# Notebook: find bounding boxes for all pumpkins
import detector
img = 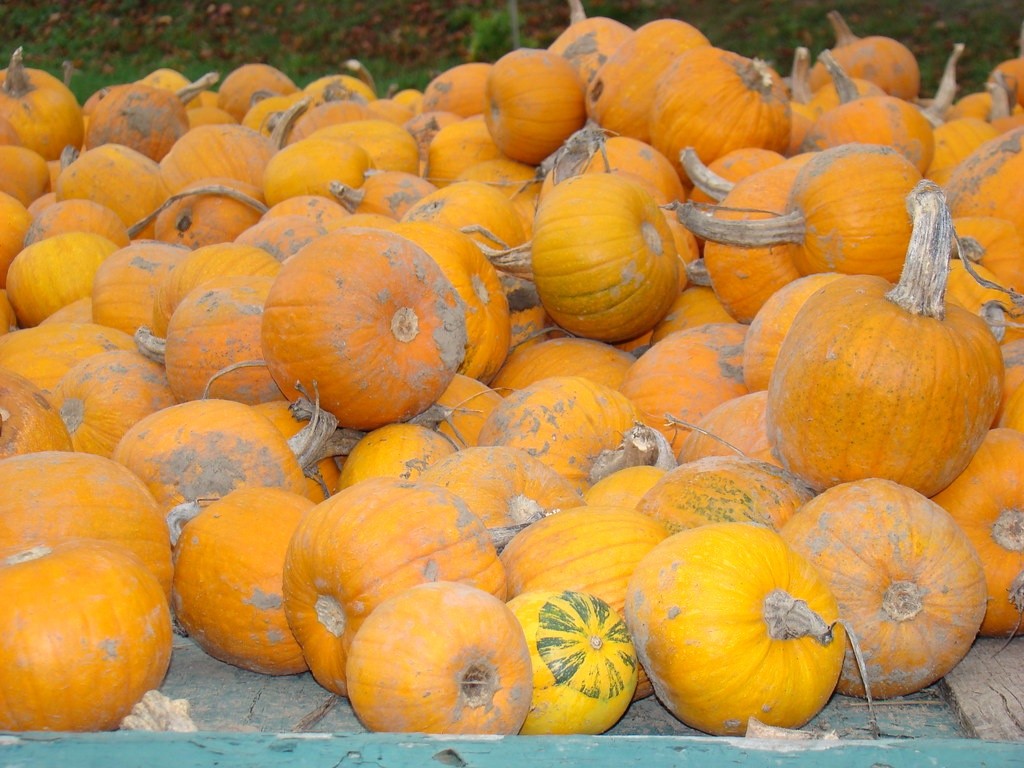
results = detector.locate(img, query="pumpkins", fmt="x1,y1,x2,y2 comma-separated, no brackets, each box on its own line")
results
0,0,1024,738
